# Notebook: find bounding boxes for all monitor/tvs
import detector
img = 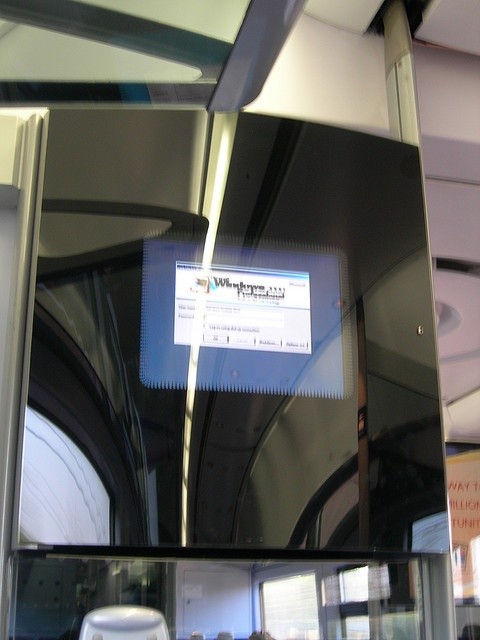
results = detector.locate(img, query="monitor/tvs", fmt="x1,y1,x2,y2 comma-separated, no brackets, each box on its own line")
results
126,225,356,403
